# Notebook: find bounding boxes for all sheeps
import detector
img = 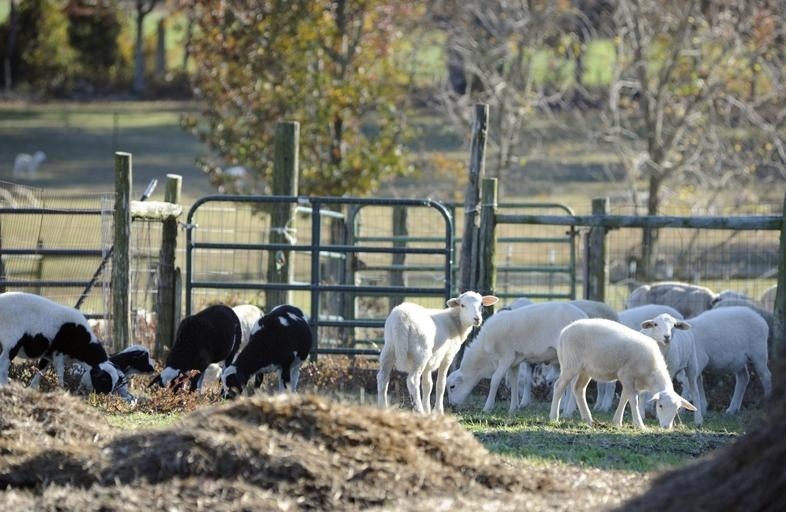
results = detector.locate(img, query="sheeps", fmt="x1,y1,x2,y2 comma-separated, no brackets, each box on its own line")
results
446,280,778,433
1,291,158,397
159,304,313,400
12,151,47,179
377,291,499,417
